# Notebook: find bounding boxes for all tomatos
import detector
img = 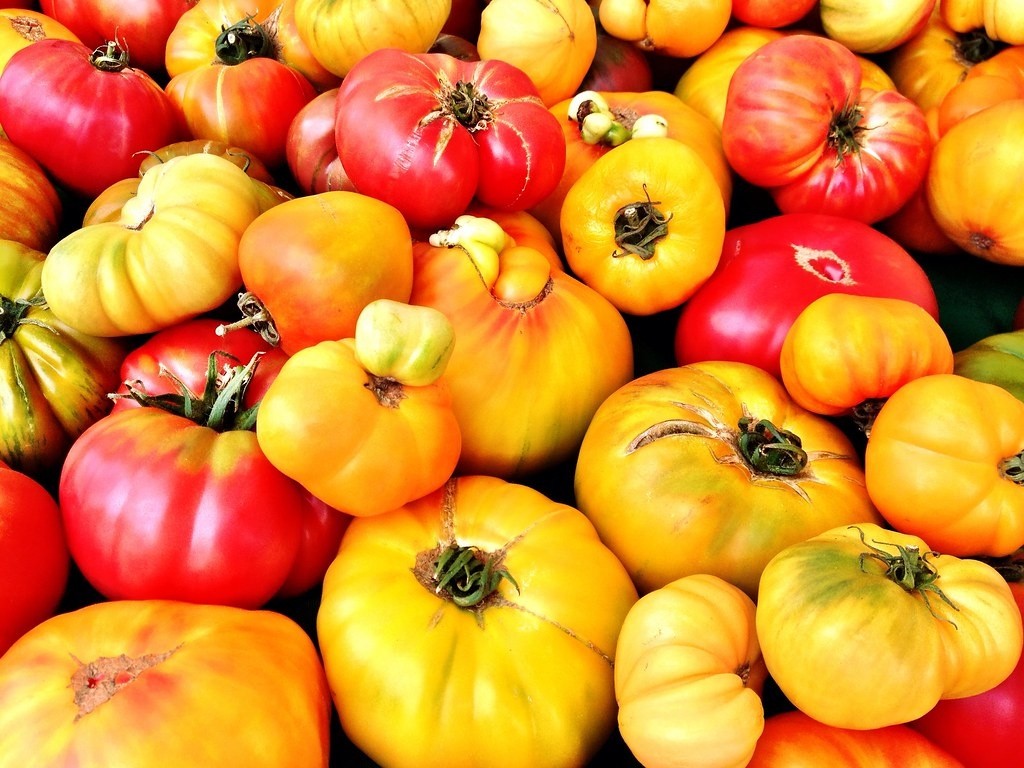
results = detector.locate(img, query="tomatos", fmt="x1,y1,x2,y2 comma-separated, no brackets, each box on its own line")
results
1,0,1024,768
